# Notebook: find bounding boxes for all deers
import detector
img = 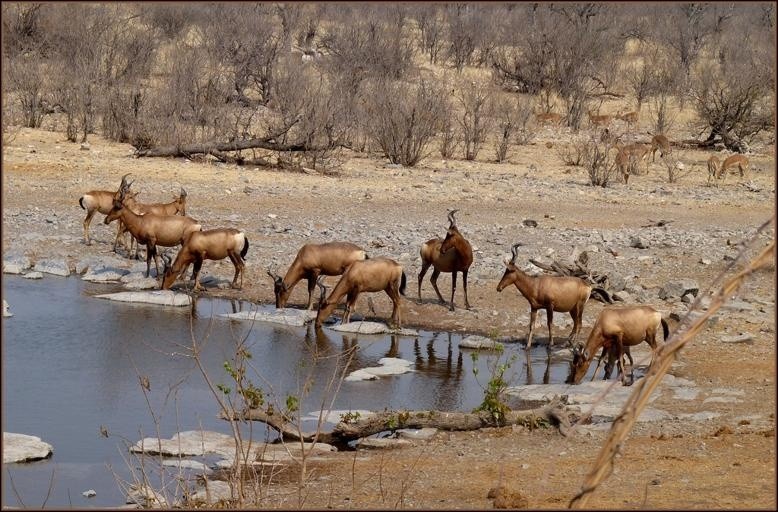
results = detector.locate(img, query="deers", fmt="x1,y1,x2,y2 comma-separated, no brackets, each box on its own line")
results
706,154,751,189
78,172,203,280
565,305,670,386
587,109,673,185
160,226,250,292
417,209,473,311
315,257,406,330
267,241,369,309
496,242,615,350
532,106,563,129
